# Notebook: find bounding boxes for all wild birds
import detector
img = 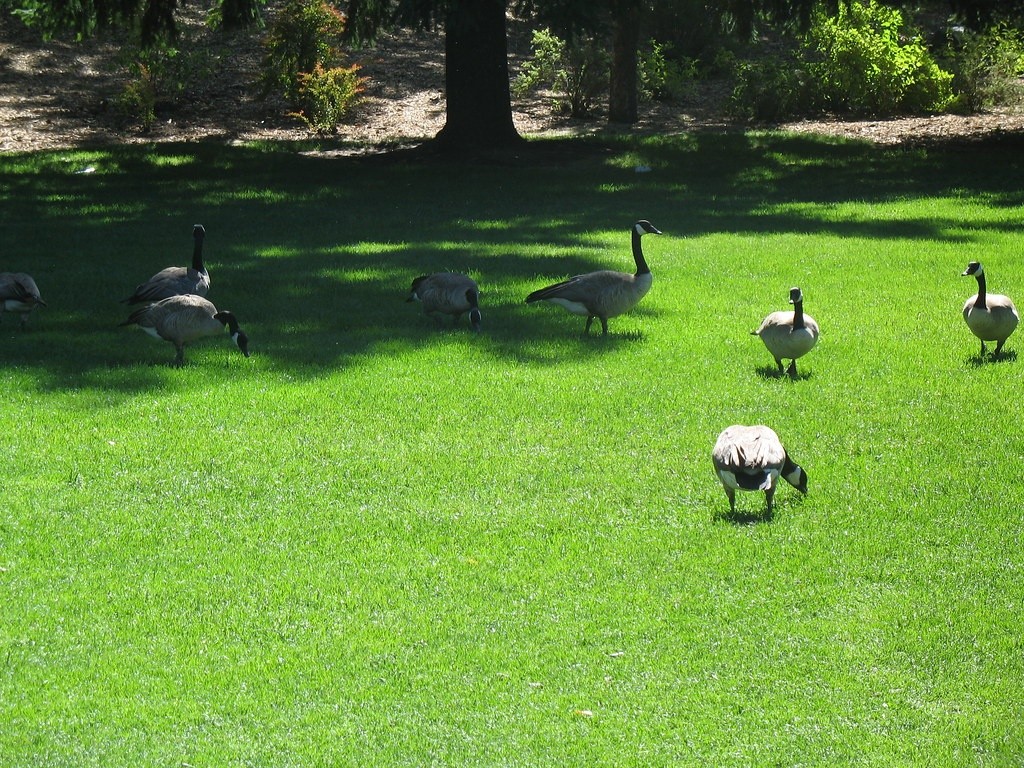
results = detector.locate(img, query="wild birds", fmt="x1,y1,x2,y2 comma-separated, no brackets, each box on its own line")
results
404,272,482,333
0,271,48,314
117,293,249,367
118,224,211,298
712,424,808,523
961,260,1016,360
750,287,819,374
526,219,662,337
